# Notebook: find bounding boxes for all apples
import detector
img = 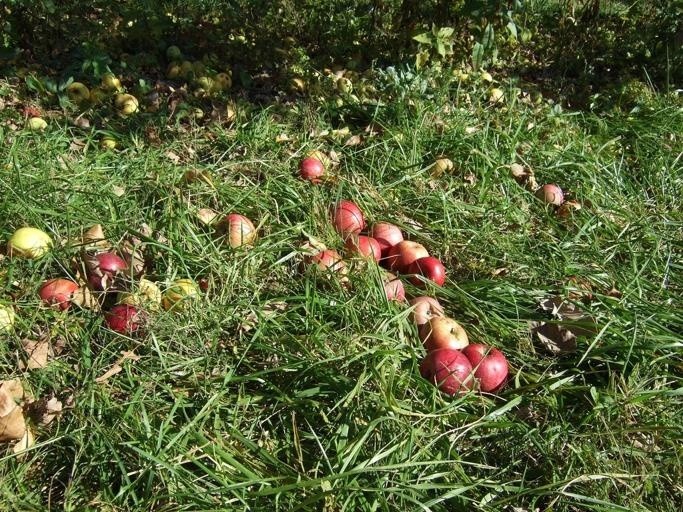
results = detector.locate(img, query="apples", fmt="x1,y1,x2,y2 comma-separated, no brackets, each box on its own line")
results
7,156,582,399
27,34,545,150
